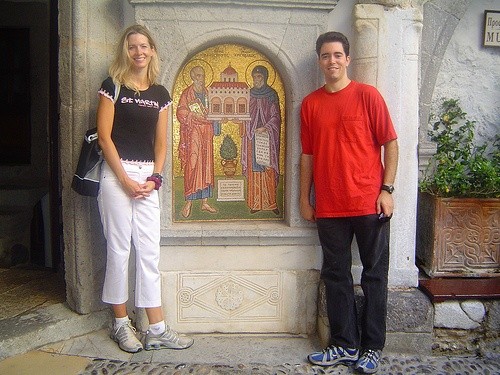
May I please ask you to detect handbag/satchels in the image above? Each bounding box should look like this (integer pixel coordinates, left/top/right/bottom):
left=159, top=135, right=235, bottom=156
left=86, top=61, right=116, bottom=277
left=71, top=127, right=103, bottom=196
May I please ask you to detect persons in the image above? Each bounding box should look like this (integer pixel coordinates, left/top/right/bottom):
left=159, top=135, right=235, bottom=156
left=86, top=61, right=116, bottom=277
left=96, top=24, right=193, bottom=354
left=299, top=31, right=399, bottom=374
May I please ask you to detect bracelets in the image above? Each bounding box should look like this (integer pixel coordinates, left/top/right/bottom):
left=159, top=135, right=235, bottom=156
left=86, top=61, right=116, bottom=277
left=147, top=173, right=163, bottom=191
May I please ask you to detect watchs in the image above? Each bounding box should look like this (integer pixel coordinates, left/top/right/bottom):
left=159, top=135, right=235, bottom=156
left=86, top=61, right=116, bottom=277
left=380, top=184, right=395, bottom=194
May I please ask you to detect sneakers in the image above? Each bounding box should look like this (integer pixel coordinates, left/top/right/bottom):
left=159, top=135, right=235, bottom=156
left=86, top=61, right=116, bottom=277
left=354, top=347, right=381, bottom=374
left=145, top=325, right=195, bottom=351
left=307, top=342, right=360, bottom=366
left=110, top=319, right=144, bottom=353
